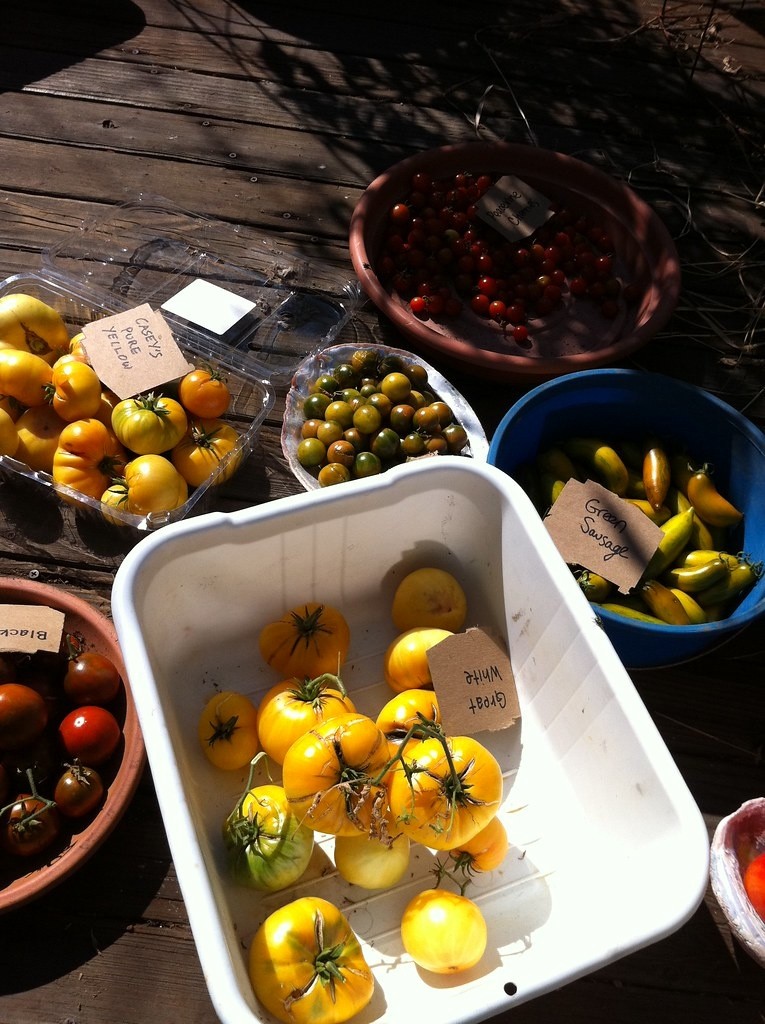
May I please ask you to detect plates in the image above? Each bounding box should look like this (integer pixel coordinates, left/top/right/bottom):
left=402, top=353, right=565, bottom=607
left=346, top=139, right=682, bottom=377
left=280, top=340, right=488, bottom=492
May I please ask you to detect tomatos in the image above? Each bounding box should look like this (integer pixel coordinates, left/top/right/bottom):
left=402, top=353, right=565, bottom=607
left=379, top=173, right=635, bottom=341
left=198, top=569, right=510, bottom=1022
left=1, top=291, right=240, bottom=521
left=1, top=632, right=119, bottom=851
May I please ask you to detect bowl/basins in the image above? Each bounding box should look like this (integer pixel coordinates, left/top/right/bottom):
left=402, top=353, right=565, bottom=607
left=482, top=367, right=764, bottom=663
left=0, top=572, right=140, bottom=914
left=713, top=794, right=765, bottom=971
left=110, top=451, right=713, bottom=1024
left=0, top=192, right=364, bottom=531
